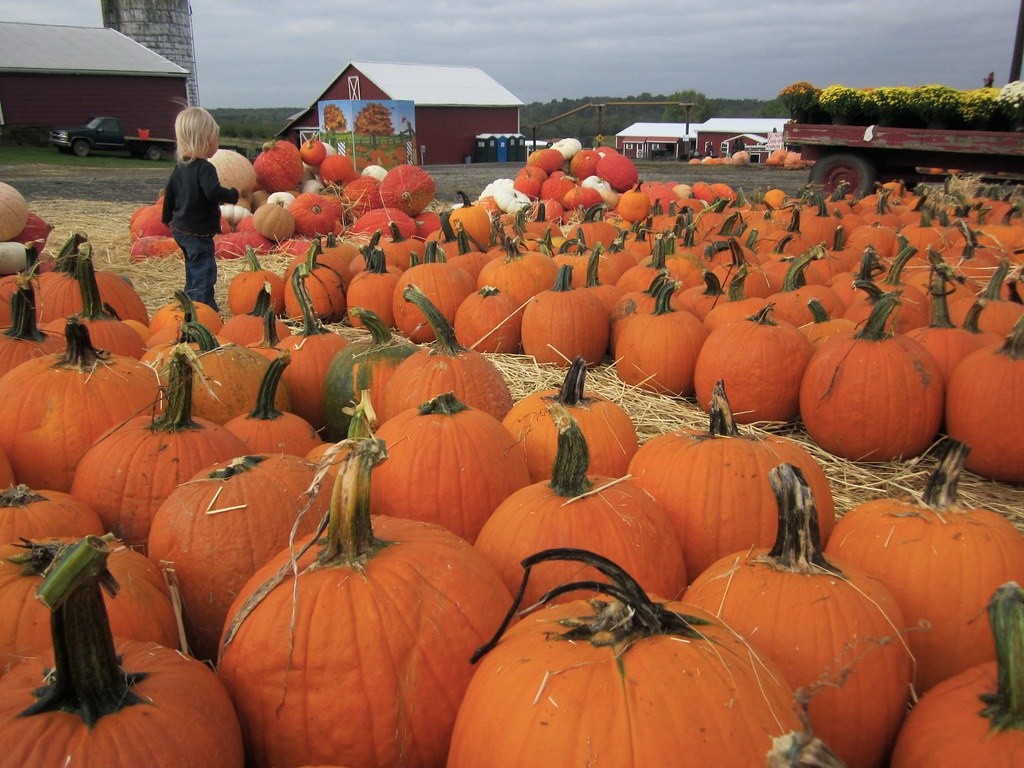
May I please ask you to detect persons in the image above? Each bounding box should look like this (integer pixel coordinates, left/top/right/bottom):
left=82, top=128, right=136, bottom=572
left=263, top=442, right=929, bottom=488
left=705, top=142, right=713, bottom=158
left=160, top=106, right=241, bottom=311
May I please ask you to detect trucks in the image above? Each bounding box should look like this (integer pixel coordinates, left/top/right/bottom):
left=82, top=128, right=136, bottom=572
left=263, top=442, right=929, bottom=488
left=777, top=122, right=1024, bottom=200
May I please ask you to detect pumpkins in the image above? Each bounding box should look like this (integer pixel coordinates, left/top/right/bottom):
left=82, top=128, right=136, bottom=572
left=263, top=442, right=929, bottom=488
left=128, top=138, right=1024, bottom=486
left=0, top=182, right=1024, bottom=768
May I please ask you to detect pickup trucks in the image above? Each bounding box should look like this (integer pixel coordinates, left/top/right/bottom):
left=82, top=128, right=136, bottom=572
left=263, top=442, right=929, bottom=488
left=47, top=114, right=177, bottom=161
